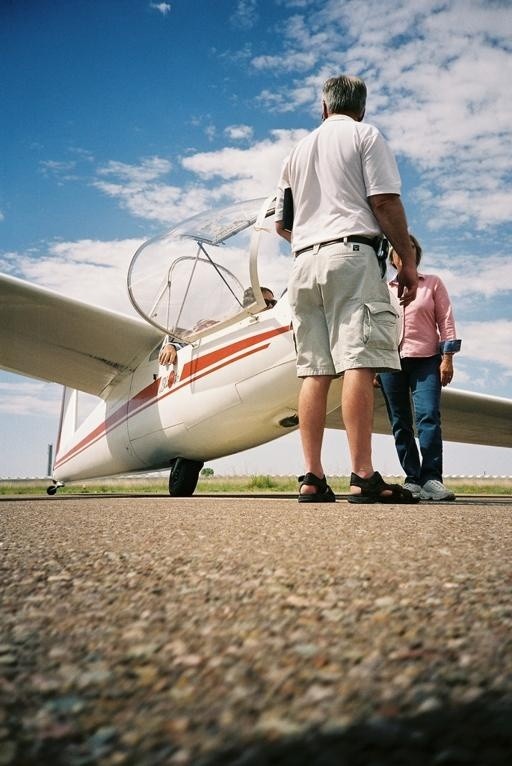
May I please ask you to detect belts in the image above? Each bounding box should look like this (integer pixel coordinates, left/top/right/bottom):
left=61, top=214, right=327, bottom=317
left=295, top=234, right=376, bottom=258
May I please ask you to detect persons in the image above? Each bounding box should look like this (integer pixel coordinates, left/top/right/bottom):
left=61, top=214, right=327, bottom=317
left=276, top=75, right=425, bottom=502
left=159, top=287, right=275, bottom=366
left=372, top=232, right=461, bottom=502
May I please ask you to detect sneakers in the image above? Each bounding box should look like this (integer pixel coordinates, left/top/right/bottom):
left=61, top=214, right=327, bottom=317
left=421, top=479, right=456, bottom=501
left=401, top=481, right=421, bottom=498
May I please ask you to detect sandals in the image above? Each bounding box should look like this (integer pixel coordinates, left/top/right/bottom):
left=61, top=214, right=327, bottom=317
left=298, top=473, right=336, bottom=502
left=346, top=471, right=420, bottom=505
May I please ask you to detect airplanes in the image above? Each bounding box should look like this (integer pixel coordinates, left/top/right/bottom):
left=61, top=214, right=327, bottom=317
left=0, top=193, right=512, bottom=499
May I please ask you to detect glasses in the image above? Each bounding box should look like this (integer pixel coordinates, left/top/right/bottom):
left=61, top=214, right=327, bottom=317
left=264, top=299, right=277, bottom=307
